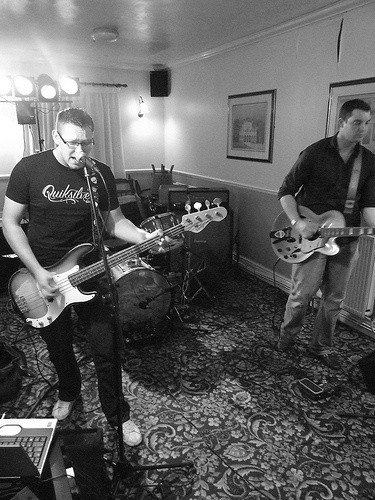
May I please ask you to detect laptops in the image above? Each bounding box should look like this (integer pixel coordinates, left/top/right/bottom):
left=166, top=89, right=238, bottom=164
left=0, top=418, right=57, bottom=481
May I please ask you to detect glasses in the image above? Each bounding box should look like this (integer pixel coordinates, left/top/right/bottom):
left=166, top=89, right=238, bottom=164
left=57, top=131, right=95, bottom=148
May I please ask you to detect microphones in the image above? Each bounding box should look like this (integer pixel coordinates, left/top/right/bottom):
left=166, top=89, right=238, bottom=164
left=76, top=152, right=100, bottom=173
left=139, top=301, right=151, bottom=309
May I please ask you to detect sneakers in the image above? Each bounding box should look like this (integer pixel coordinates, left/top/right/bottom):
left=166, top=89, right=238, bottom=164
left=52, top=391, right=81, bottom=420
left=115, top=419, right=142, bottom=446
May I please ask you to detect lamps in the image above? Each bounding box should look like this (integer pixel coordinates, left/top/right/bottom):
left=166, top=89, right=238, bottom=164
left=37, top=74, right=59, bottom=101
left=138, top=96, right=150, bottom=118
left=60, top=77, right=80, bottom=96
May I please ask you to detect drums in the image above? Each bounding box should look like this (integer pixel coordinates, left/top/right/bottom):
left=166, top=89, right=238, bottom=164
left=109, top=257, right=173, bottom=323
left=139, top=212, right=185, bottom=254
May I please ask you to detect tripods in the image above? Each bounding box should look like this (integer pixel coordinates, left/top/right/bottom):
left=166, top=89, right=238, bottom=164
left=84, top=164, right=193, bottom=500
left=178, top=234, right=215, bottom=313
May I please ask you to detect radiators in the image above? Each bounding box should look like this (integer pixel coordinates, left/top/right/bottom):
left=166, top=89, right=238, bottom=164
left=316, top=234, right=375, bottom=319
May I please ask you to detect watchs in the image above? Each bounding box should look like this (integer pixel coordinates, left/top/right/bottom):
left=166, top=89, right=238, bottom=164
left=291, top=219, right=297, bottom=226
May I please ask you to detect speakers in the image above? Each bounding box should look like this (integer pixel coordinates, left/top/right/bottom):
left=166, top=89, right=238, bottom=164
left=150, top=71, right=169, bottom=97
left=167, top=209, right=234, bottom=273
left=15, top=102, right=36, bottom=124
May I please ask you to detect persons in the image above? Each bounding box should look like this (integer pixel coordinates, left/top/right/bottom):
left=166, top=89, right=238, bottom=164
left=275, top=99, right=375, bottom=369
left=1, top=108, right=174, bottom=446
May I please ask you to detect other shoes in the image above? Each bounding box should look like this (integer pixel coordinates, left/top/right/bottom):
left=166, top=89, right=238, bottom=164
left=313, top=352, right=341, bottom=371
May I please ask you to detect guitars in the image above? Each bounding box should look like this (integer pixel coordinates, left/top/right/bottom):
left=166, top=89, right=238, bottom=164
left=7, top=197, right=227, bottom=329
left=268, top=205, right=374, bottom=264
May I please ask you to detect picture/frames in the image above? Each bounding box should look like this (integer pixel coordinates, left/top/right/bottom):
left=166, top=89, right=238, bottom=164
left=226, top=88, right=277, bottom=163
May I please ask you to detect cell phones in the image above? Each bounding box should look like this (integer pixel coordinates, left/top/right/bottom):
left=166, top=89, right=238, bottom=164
left=299, top=378, right=324, bottom=394
